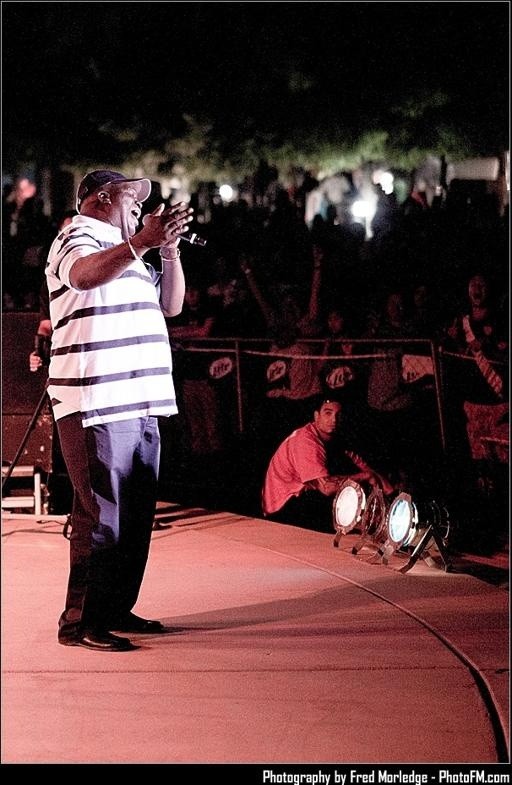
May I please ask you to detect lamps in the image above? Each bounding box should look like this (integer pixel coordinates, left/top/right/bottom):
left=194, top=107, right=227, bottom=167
left=330, top=472, right=455, bottom=577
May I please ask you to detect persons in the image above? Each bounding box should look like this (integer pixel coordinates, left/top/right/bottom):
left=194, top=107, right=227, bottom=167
left=2, top=167, right=508, bottom=651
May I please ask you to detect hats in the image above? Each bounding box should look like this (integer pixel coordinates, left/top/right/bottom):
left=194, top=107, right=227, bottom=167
left=76, top=169, right=152, bottom=215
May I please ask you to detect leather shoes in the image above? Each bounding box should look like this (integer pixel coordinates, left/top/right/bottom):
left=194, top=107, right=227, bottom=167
left=109, top=611, right=161, bottom=633
left=74, top=628, right=130, bottom=651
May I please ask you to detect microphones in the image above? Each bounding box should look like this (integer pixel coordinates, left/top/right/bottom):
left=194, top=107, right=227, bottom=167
left=142, top=214, right=207, bottom=247
